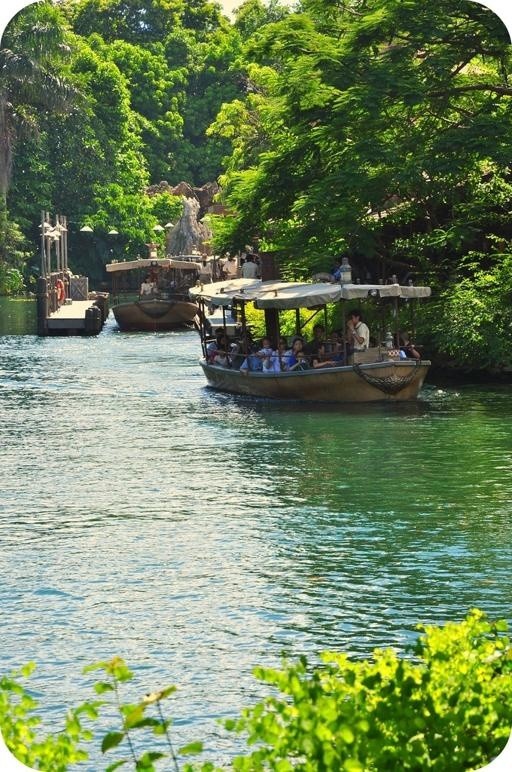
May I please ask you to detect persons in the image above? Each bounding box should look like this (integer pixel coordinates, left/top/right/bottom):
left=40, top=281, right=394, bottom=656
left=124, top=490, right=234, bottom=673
left=240, top=256, right=259, bottom=278
left=139, top=275, right=157, bottom=295
left=206, top=311, right=376, bottom=375
left=223, top=259, right=236, bottom=279
left=198, top=253, right=213, bottom=285
left=399, top=331, right=421, bottom=358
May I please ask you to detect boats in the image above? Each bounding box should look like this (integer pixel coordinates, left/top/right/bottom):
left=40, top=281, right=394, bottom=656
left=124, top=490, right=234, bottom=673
left=186, top=275, right=432, bottom=404
left=105, top=257, right=203, bottom=332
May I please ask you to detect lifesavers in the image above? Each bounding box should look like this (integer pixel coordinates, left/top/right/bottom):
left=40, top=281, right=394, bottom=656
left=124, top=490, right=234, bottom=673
left=57, top=280, right=65, bottom=301
